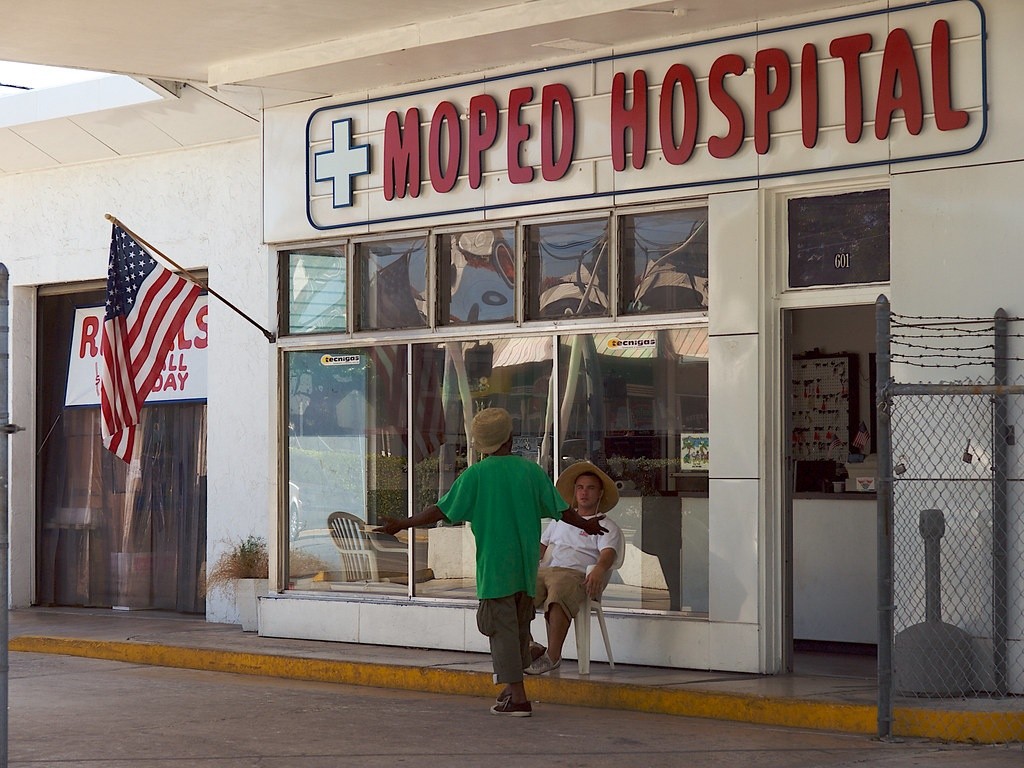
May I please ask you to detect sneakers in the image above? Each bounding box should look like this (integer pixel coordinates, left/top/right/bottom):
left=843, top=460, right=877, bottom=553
left=490, top=695, right=532, bottom=716
left=496, top=688, right=512, bottom=704
left=523, top=642, right=547, bottom=669
left=523, top=651, right=562, bottom=675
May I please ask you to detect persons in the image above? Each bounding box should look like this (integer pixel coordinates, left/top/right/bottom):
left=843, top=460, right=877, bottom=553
left=523, top=461, right=620, bottom=675
left=370, top=407, right=609, bottom=717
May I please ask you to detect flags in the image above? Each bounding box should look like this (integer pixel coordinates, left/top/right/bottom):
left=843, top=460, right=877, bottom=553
left=852, top=422, right=870, bottom=451
left=359, top=255, right=447, bottom=464
left=100, top=224, right=202, bottom=466
left=830, top=434, right=842, bottom=452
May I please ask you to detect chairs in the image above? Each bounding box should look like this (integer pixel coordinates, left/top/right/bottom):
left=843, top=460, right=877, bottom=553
left=574, top=528, right=626, bottom=674
left=327, top=511, right=408, bottom=583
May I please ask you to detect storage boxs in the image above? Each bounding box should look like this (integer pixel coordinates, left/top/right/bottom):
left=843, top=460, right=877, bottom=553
left=846, top=477, right=878, bottom=491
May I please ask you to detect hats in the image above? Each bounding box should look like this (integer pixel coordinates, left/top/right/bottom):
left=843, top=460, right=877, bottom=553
left=471, top=408, right=512, bottom=453
left=556, top=462, right=619, bottom=513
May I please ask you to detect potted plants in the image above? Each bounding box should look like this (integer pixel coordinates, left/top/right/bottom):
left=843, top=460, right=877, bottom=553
left=198, top=530, right=268, bottom=632
